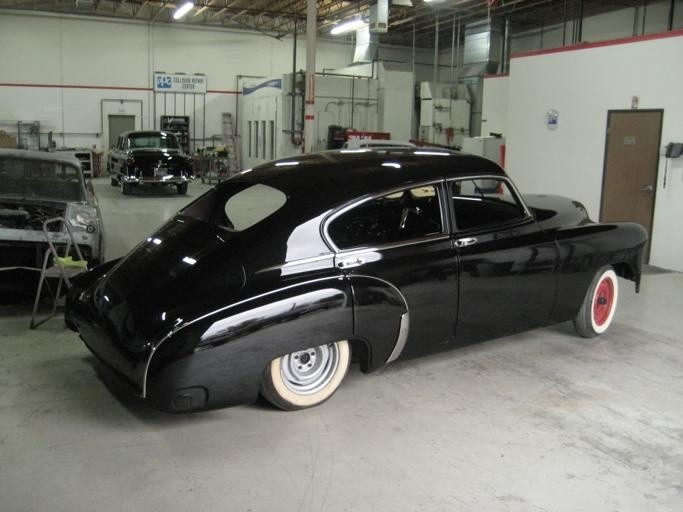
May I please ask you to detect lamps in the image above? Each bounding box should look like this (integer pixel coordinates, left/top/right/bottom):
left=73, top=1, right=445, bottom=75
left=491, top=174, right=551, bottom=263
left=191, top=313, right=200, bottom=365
left=330, top=16, right=370, bottom=36
left=171, top=1, right=194, bottom=22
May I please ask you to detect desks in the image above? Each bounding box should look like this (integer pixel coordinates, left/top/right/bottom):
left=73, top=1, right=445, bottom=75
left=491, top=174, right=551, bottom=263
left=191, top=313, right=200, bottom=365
left=200, top=158, right=229, bottom=185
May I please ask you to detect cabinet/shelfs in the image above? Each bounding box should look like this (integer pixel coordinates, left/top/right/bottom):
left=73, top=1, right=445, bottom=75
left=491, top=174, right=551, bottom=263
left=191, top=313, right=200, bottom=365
left=74, top=152, right=93, bottom=179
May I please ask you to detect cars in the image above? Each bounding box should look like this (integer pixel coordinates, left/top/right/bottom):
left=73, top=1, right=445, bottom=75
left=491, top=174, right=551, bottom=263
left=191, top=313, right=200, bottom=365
left=108, top=130, right=194, bottom=199
left=63, top=143, right=650, bottom=416
left=0, top=145, right=107, bottom=311
